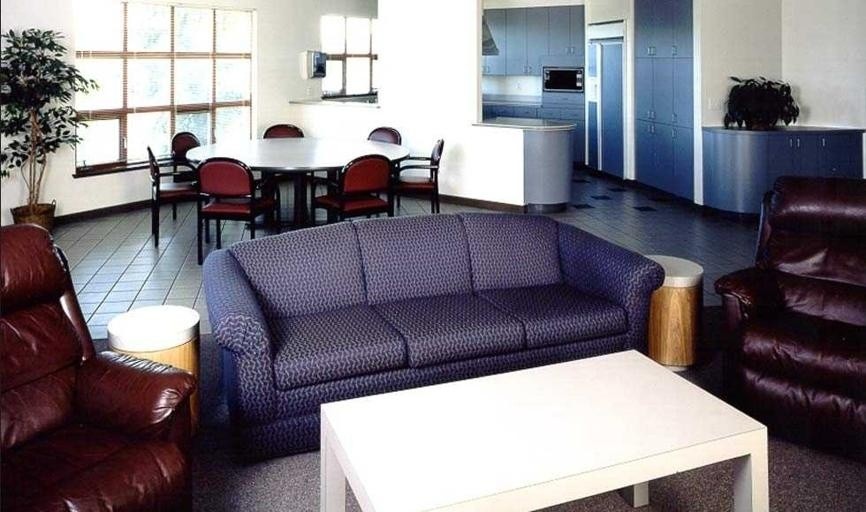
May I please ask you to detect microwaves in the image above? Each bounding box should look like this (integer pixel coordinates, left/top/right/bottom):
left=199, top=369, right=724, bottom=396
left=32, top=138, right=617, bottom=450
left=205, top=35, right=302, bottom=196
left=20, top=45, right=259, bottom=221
left=543, top=68, right=584, bottom=92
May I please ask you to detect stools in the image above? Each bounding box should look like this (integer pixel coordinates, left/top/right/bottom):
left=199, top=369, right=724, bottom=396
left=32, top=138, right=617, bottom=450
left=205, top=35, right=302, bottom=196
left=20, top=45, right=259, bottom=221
left=105, top=304, right=202, bottom=432
left=641, top=254, right=705, bottom=372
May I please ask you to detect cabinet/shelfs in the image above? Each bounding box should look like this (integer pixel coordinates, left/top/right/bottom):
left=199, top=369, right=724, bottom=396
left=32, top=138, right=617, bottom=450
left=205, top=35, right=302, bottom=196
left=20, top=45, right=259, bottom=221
left=549, top=7, right=584, bottom=56
left=484, top=105, right=537, bottom=120
left=635, top=119, right=673, bottom=196
left=672, top=60, right=694, bottom=127
left=702, top=128, right=864, bottom=215
left=635, top=58, right=671, bottom=125
left=506, top=8, right=548, bottom=75
left=538, top=109, right=584, bottom=164
left=481, top=10, right=505, bottom=75
left=671, top=1, right=692, bottom=59
left=635, top=1, right=672, bottom=57
left=673, top=127, right=695, bottom=200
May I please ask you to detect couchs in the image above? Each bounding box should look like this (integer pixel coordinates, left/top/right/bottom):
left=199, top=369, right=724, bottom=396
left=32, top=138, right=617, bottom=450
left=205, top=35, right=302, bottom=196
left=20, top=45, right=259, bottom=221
left=200, top=213, right=666, bottom=464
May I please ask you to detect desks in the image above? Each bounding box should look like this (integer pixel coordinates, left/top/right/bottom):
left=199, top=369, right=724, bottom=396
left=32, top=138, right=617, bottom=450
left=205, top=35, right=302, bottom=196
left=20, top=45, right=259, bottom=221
left=185, top=137, right=410, bottom=232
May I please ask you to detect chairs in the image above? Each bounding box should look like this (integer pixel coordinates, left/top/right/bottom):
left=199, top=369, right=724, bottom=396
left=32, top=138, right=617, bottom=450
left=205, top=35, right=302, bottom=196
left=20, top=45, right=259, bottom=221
left=1, top=220, right=197, bottom=512
left=712, top=177, right=865, bottom=455
left=308, top=154, right=396, bottom=226
left=171, top=131, right=201, bottom=221
left=395, top=139, right=445, bottom=214
left=260, top=124, right=307, bottom=215
left=194, top=157, right=279, bottom=267
left=144, top=146, right=210, bottom=248
left=368, top=127, right=403, bottom=218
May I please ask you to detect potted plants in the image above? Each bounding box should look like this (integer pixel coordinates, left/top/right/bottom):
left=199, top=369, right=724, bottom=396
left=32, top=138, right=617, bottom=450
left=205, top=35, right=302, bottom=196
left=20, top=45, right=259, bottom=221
left=723, top=76, right=799, bottom=130
left=0, top=27, right=101, bottom=234
